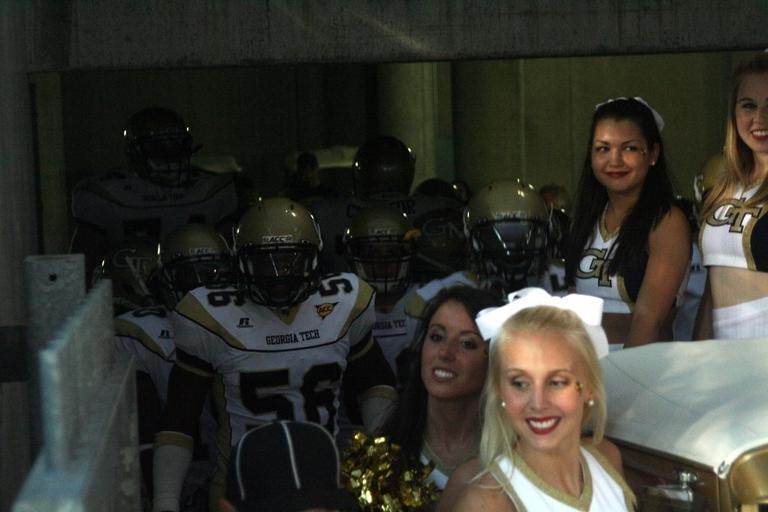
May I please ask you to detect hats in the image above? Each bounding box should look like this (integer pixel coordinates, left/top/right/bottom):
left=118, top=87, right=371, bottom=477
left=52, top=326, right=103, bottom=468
left=223, top=419, right=353, bottom=512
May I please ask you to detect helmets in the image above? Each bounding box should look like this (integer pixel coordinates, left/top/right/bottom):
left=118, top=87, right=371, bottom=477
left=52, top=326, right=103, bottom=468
left=406, top=179, right=466, bottom=265
left=462, top=181, right=551, bottom=285
left=231, top=197, right=323, bottom=310
left=90, top=240, right=177, bottom=313
left=351, top=135, right=415, bottom=201
left=341, top=192, right=414, bottom=306
left=121, top=108, right=194, bottom=187
left=142, top=225, right=233, bottom=312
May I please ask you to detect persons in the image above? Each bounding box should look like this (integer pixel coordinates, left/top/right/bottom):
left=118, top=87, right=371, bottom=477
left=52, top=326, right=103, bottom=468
left=568, top=97, right=690, bottom=354
left=400, top=283, right=639, bottom=511
left=71, top=107, right=575, bottom=512
left=692, top=54, right=766, bottom=342
